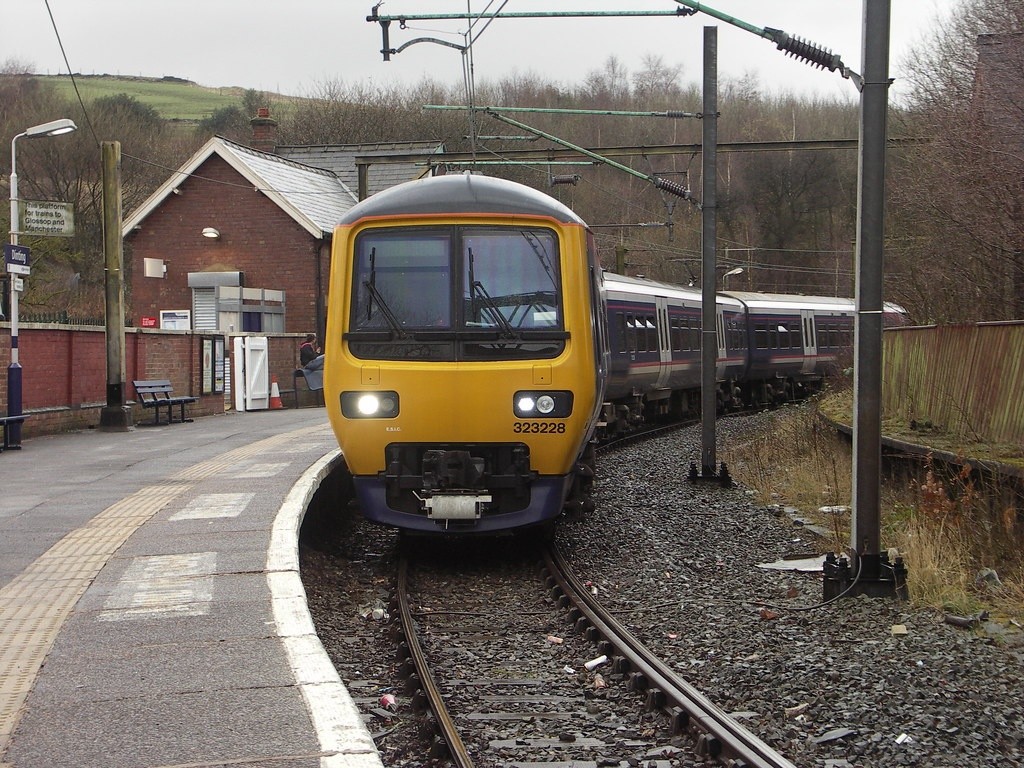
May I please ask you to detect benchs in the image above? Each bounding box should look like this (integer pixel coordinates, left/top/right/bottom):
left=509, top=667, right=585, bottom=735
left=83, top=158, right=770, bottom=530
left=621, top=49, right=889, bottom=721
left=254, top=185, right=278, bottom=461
left=133, top=379, right=196, bottom=426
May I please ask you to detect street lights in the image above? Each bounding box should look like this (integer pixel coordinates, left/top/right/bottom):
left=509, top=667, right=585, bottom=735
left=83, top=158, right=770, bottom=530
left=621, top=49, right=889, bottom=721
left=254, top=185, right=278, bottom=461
left=2, top=118, right=76, bottom=456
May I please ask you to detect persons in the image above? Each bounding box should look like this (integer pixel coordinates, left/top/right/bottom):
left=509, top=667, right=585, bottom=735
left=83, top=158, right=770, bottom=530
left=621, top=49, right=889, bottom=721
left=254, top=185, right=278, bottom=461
left=300, top=334, right=321, bottom=367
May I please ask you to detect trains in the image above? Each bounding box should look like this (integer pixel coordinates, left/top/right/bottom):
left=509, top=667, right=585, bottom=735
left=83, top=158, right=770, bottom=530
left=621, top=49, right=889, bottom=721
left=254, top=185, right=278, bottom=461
left=325, top=170, right=926, bottom=560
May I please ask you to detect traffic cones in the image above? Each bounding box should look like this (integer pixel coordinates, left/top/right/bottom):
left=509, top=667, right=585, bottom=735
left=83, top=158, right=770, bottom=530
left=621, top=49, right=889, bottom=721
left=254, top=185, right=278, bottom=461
left=265, top=375, right=287, bottom=410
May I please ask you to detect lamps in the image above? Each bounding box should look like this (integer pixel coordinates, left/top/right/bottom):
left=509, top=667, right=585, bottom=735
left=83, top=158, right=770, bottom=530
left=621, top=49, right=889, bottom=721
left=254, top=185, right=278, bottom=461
left=202, top=227, right=220, bottom=238
